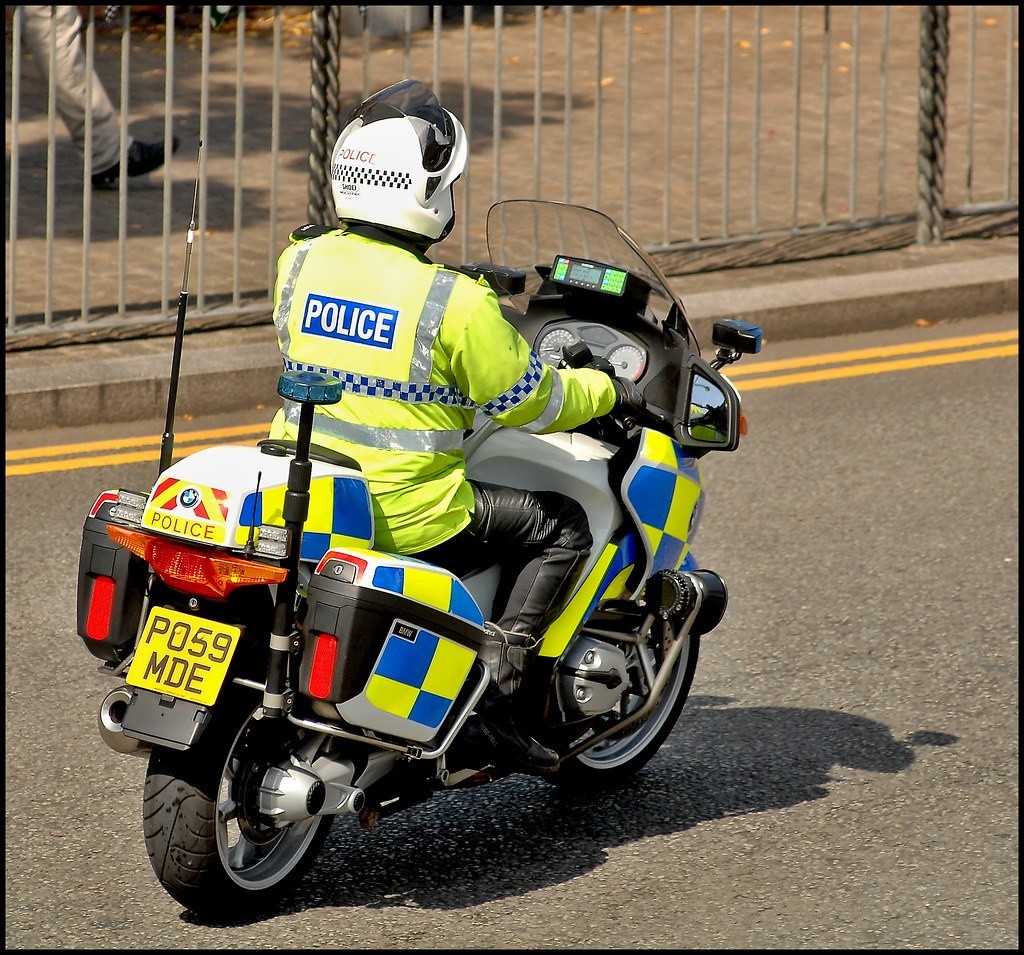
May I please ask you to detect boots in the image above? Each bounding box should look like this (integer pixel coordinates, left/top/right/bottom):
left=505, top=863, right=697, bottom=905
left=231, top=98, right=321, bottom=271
left=463, top=619, right=560, bottom=775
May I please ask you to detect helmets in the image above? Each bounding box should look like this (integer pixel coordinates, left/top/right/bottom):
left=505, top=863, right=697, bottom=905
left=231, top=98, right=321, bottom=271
left=330, top=104, right=469, bottom=246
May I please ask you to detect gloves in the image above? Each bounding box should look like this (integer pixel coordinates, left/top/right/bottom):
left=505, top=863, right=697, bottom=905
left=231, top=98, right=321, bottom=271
left=610, top=376, right=646, bottom=414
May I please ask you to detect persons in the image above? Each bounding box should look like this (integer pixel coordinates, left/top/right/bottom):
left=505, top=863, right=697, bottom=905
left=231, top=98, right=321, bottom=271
left=268, top=79, right=643, bottom=772
left=11, top=6, right=180, bottom=192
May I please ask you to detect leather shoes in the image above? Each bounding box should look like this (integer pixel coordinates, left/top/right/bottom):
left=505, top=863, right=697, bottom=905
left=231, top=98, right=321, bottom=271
left=91, top=137, right=178, bottom=188
